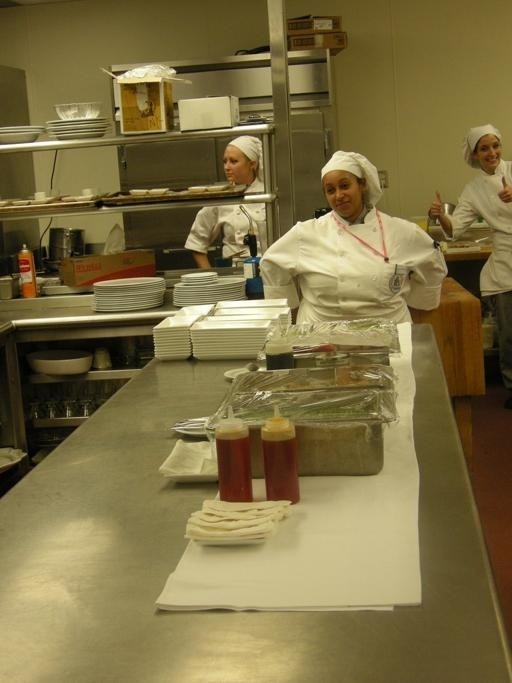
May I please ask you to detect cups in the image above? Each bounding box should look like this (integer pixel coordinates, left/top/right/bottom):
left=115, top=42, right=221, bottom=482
left=92, top=346, right=113, bottom=369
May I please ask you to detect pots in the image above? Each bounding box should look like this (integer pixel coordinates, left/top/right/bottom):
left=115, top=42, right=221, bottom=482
left=47, top=227, right=85, bottom=261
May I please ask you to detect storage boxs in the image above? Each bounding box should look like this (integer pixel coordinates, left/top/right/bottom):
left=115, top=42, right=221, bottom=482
left=178, top=95, right=239, bottom=132
left=99, top=64, right=192, bottom=135
left=59, top=247, right=156, bottom=289
left=287, top=13, right=348, bottom=53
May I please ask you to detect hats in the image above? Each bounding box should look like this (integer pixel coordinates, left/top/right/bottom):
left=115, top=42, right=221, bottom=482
left=229, top=135, right=264, bottom=183
left=462, top=123, right=503, bottom=169
left=320, top=150, right=383, bottom=210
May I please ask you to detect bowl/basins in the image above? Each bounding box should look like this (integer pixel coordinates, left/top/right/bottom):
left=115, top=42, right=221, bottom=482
left=54, top=101, right=102, bottom=120
left=25, top=350, right=93, bottom=375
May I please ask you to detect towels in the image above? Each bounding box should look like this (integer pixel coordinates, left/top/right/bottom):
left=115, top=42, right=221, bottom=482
left=152, top=317, right=424, bottom=612
left=158, top=440, right=218, bottom=479
left=185, top=498, right=294, bottom=543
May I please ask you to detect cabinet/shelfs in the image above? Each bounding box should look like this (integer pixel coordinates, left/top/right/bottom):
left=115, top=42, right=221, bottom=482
left=0, top=122, right=277, bottom=247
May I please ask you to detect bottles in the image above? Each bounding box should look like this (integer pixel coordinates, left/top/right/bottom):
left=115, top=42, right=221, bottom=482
left=481, top=312, right=497, bottom=349
left=261, top=404, right=301, bottom=506
left=265, top=329, right=295, bottom=369
left=17, top=244, right=38, bottom=298
left=214, top=405, right=253, bottom=503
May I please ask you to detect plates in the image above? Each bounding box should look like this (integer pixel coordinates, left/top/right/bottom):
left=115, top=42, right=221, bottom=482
left=0, top=195, right=56, bottom=206
left=174, top=417, right=208, bottom=438
left=0, top=126, right=46, bottom=144
left=152, top=298, right=293, bottom=363
left=224, top=366, right=266, bottom=379
left=172, top=271, right=248, bottom=307
left=46, top=117, right=112, bottom=140
left=187, top=183, right=231, bottom=192
left=160, top=438, right=218, bottom=484
left=129, top=187, right=169, bottom=196
left=0, top=447, right=28, bottom=474
left=42, top=285, right=91, bottom=295
left=92, top=276, right=166, bottom=312
left=61, top=194, right=102, bottom=201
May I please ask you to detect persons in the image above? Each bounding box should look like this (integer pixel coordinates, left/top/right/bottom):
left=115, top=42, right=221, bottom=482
left=184, top=132, right=268, bottom=269
left=259, top=149, right=447, bottom=328
left=429, top=122, right=512, bottom=412
